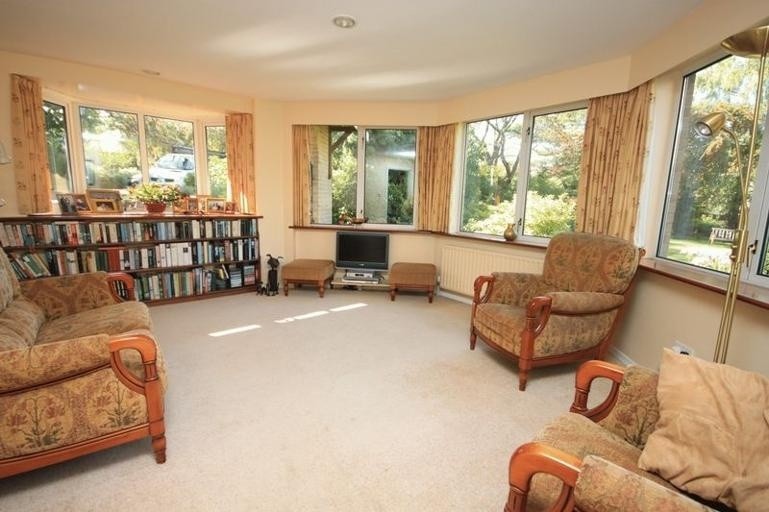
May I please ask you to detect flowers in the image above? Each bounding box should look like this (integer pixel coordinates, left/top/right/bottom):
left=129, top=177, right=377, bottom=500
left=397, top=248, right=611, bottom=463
left=127, top=182, right=183, bottom=203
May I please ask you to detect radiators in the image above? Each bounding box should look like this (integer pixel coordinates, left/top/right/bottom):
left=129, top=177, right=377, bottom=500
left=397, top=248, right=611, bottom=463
left=439, top=244, right=545, bottom=300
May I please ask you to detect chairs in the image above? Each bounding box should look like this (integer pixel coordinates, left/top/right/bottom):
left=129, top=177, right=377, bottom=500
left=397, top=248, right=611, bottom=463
left=503, top=361, right=768, bottom=512
left=470, top=231, right=647, bottom=391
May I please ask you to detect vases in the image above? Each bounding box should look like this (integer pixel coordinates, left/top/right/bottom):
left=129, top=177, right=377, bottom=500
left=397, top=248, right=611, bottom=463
left=144, top=200, right=166, bottom=213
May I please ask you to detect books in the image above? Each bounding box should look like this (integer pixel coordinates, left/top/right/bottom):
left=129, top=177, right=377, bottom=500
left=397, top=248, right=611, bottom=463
left=1, top=217, right=259, bottom=303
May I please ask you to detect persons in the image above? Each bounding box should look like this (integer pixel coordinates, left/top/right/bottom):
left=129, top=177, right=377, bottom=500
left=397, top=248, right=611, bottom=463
left=215, top=203, right=221, bottom=210
left=96, top=202, right=107, bottom=212
left=212, top=204, right=218, bottom=210
left=60, top=197, right=76, bottom=214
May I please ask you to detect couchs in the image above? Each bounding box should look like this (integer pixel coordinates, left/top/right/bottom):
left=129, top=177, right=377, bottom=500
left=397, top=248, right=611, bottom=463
left=0, top=247, right=170, bottom=479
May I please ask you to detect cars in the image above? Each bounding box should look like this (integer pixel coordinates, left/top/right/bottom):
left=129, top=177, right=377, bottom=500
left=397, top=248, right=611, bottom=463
left=128, top=173, right=143, bottom=185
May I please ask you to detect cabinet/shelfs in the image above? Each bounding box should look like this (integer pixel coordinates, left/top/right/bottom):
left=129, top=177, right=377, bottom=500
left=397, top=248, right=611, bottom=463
left=1, top=213, right=268, bottom=309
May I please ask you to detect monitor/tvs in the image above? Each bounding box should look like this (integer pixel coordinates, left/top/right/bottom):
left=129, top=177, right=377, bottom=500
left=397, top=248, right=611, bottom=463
left=336, top=232, right=390, bottom=272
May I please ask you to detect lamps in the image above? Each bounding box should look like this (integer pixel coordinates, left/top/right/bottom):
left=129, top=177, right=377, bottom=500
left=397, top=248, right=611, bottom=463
left=695, top=22, right=768, bottom=362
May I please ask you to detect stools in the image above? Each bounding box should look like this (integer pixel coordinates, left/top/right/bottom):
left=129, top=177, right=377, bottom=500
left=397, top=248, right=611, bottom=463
left=280, top=257, right=335, bottom=297
left=391, top=263, right=436, bottom=302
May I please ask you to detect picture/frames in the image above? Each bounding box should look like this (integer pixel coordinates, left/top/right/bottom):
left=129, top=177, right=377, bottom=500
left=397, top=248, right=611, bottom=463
left=56, top=188, right=123, bottom=215
left=172, top=195, right=235, bottom=214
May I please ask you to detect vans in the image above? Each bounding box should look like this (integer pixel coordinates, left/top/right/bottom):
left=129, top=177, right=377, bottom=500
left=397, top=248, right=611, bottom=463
left=148, top=154, right=195, bottom=188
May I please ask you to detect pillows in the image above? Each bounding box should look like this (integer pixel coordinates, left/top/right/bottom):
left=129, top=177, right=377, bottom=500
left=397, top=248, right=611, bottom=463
left=637, top=346, right=768, bottom=512
